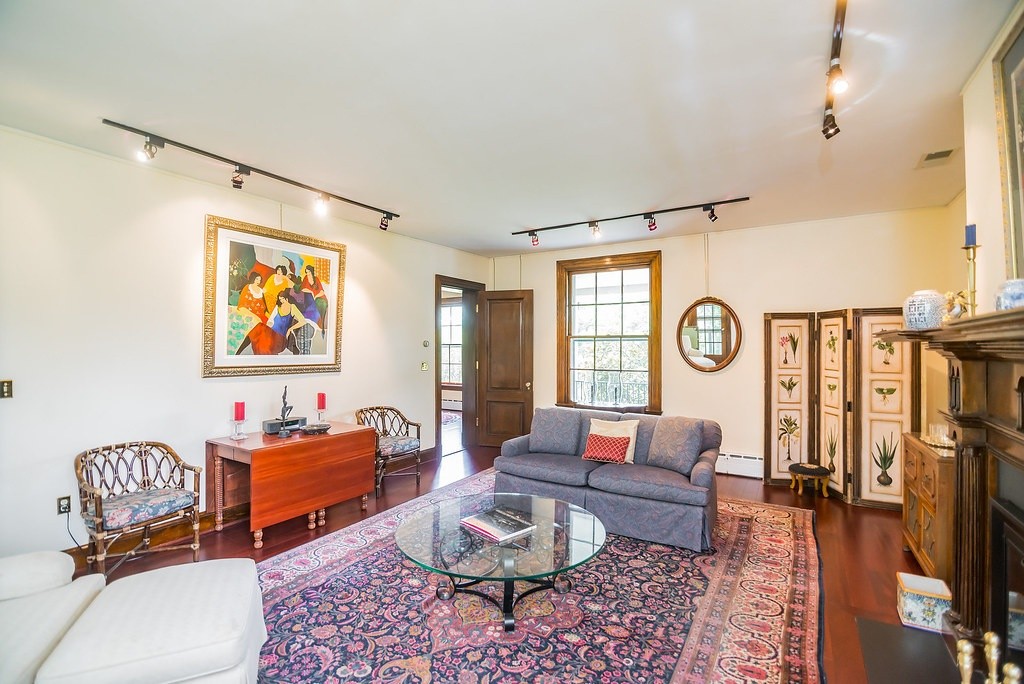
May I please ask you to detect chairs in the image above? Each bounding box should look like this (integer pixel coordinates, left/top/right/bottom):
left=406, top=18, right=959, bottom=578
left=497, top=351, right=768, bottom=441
left=0, top=548, right=270, bottom=684
left=73, top=441, right=202, bottom=579
left=355, top=406, right=423, bottom=499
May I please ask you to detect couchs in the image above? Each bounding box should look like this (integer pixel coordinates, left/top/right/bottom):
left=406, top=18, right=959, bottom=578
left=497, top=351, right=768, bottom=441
left=493, top=409, right=724, bottom=553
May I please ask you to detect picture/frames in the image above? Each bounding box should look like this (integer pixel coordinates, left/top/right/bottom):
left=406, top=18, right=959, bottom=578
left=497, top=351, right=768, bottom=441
left=200, top=215, right=348, bottom=378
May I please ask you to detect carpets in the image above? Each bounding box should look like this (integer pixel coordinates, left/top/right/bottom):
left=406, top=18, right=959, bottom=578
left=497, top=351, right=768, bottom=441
left=855, top=616, right=961, bottom=684
left=256, top=465, right=828, bottom=684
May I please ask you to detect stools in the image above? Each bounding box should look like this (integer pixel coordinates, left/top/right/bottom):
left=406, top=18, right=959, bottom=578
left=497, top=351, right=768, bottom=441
left=788, top=462, right=832, bottom=500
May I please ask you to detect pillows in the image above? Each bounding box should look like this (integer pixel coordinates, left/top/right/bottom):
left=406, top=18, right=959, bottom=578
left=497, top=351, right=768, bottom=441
left=582, top=434, right=631, bottom=466
left=646, top=416, right=705, bottom=478
left=527, top=406, right=582, bottom=456
left=582, top=417, right=640, bottom=465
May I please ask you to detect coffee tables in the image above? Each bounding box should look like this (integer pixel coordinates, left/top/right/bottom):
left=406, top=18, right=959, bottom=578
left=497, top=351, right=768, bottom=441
left=393, top=492, right=607, bottom=633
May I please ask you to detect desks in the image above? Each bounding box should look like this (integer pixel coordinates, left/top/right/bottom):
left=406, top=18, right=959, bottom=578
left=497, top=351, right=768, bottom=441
left=204, top=420, right=376, bottom=550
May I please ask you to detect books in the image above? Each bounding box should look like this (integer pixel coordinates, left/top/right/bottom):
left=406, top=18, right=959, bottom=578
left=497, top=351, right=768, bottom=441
left=460, top=509, right=537, bottom=542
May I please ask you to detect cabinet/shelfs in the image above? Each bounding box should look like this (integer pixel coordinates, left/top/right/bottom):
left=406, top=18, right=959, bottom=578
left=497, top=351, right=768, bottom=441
left=901, top=431, right=956, bottom=580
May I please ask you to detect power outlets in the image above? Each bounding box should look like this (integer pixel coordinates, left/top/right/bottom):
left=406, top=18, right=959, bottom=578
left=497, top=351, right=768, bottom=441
left=57, top=496, right=70, bottom=515
left=0, top=380, right=12, bottom=398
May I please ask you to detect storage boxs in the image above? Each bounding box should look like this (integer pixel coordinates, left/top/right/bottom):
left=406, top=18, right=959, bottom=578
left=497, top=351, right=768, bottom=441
left=1008, top=591, right=1024, bottom=652
left=894, top=571, right=956, bottom=636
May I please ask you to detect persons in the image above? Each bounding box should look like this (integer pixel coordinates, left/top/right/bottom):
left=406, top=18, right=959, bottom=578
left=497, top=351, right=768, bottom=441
left=276, top=386, right=292, bottom=420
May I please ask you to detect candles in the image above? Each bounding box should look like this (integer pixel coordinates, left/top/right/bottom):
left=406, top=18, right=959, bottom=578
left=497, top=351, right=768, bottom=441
left=964, top=224, right=977, bottom=246
left=317, top=393, right=326, bottom=409
left=234, top=402, right=246, bottom=421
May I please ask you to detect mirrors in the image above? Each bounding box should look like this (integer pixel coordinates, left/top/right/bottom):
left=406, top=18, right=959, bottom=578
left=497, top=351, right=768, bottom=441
left=676, top=296, right=742, bottom=374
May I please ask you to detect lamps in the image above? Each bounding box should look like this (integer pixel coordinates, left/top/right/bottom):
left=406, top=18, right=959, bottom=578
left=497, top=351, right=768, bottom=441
left=828, top=67, right=848, bottom=94
left=703, top=205, right=718, bottom=223
left=135, top=137, right=165, bottom=162
left=643, top=213, right=657, bottom=232
left=589, top=222, right=601, bottom=238
left=230, top=166, right=251, bottom=189
left=528, top=231, right=539, bottom=246
left=379, top=213, right=393, bottom=231
left=313, top=194, right=330, bottom=218
left=822, top=114, right=841, bottom=140
